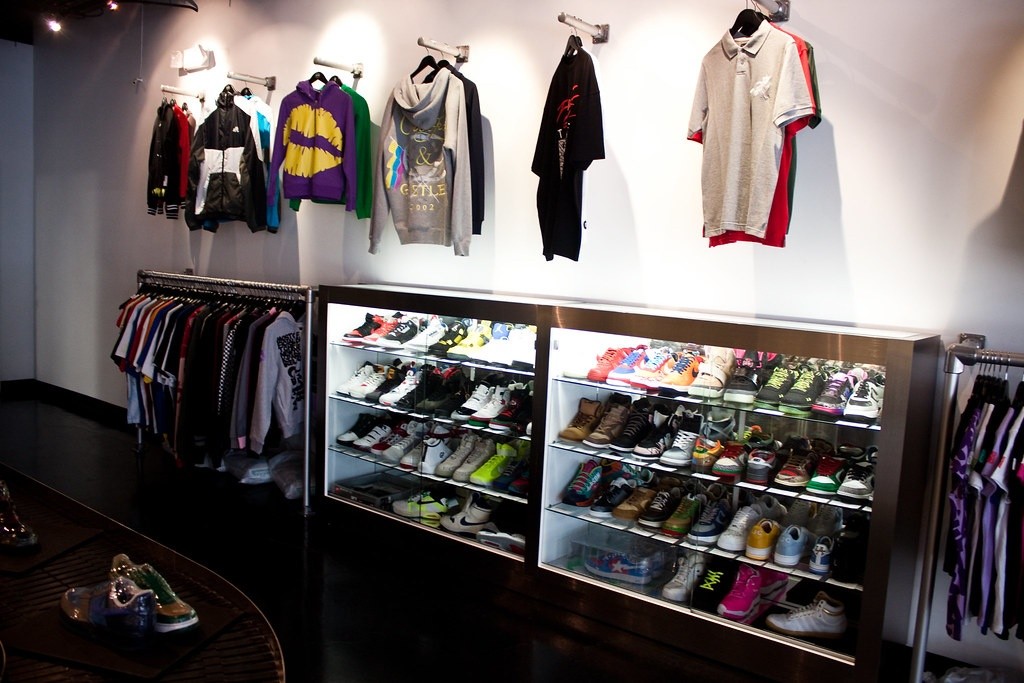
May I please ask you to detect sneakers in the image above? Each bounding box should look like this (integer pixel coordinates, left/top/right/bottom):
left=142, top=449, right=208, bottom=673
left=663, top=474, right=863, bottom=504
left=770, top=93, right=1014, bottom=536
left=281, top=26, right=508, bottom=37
left=746, top=516, right=786, bottom=561
left=339, top=360, right=533, bottom=552
left=719, top=563, right=789, bottom=619
left=561, top=455, right=678, bottom=522
left=341, top=305, right=536, bottom=370
left=810, top=533, right=837, bottom=575
left=809, top=503, right=843, bottom=534
left=109, top=554, right=198, bottom=632
left=686, top=496, right=734, bottom=546
left=686, top=555, right=742, bottom=610
left=782, top=499, right=819, bottom=527
left=716, top=501, right=764, bottom=552
left=657, top=493, right=704, bottom=538
left=753, top=493, right=786, bottom=521
left=584, top=543, right=671, bottom=584
left=638, top=490, right=680, bottom=527
left=774, top=524, right=815, bottom=566
left=62, top=578, right=156, bottom=631
left=556, top=344, right=886, bottom=500
left=660, top=552, right=707, bottom=601
left=766, top=591, right=847, bottom=635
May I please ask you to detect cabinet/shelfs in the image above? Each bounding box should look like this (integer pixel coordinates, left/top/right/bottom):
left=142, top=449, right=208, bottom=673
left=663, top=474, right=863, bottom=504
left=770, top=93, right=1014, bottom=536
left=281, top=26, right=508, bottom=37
left=311, top=284, right=939, bottom=683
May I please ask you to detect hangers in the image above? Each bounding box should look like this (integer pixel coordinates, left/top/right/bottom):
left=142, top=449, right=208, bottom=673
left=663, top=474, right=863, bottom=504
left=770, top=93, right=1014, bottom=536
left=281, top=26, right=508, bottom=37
left=308, top=57, right=343, bottom=88
left=224, top=73, right=254, bottom=98
left=730, top=0, right=769, bottom=36
left=160, top=86, right=190, bottom=113
left=970, top=351, right=1024, bottom=409
left=119, top=275, right=306, bottom=322
left=410, top=39, right=451, bottom=83
left=562, top=14, right=584, bottom=57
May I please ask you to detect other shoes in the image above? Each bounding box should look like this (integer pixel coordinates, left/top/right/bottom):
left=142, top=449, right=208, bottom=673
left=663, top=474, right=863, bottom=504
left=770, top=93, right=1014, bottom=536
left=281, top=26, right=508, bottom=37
left=0, top=480, right=38, bottom=545
left=705, top=483, right=732, bottom=502
left=827, top=511, right=871, bottom=581
left=679, top=477, right=705, bottom=493
